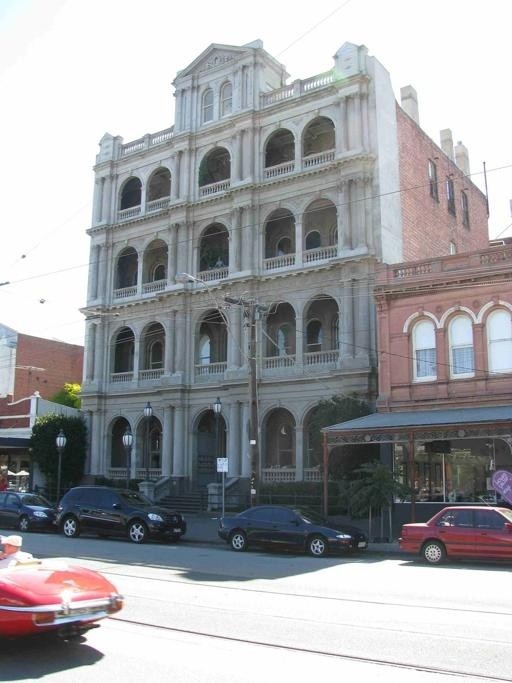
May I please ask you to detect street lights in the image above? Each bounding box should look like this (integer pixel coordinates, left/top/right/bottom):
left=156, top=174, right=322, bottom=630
left=143, top=401, right=152, bottom=481
left=122, top=425, right=133, bottom=488
left=174, top=271, right=262, bottom=507
left=213, top=397, right=221, bottom=483
left=55, top=428, right=67, bottom=507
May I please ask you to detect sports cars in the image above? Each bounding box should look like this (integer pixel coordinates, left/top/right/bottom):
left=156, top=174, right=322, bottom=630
left=1, top=536, right=123, bottom=641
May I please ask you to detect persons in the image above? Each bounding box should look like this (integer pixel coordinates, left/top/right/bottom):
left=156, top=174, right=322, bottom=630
left=214, top=255, right=226, bottom=269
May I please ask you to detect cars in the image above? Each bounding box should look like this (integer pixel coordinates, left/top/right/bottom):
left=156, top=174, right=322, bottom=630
left=399, top=505, right=512, bottom=564
left=218, top=503, right=369, bottom=557
left=55, top=485, right=186, bottom=544
left=0, top=489, right=59, bottom=531
